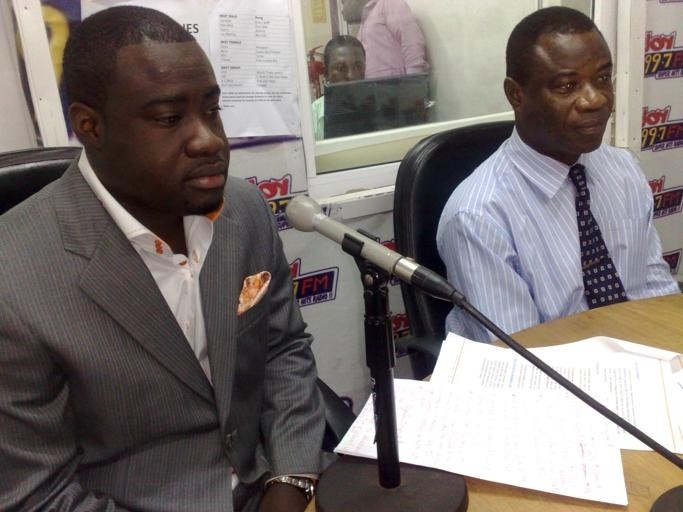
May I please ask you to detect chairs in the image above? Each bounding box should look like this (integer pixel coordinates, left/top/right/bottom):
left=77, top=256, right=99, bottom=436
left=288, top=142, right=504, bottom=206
left=0, top=145, right=358, bottom=466
left=392, top=120, right=519, bottom=380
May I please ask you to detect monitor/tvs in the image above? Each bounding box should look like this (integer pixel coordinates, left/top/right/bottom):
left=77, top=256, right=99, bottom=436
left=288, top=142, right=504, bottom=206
left=323, top=72, right=431, bottom=140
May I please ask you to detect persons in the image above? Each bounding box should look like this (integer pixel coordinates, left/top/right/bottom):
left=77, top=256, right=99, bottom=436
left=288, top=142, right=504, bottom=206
left=0, top=7, right=329, bottom=512
left=437, top=5, right=682, bottom=342
left=341, top=0, right=430, bottom=80
left=311, top=35, right=366, bottom=141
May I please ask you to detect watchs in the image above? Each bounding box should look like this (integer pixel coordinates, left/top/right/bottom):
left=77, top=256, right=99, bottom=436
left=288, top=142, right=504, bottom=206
left=267, top=475, right=314, bottom=501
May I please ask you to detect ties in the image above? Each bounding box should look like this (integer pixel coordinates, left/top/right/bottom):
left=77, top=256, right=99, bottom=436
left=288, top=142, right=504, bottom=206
left=568, top=164, right=627, bottom=310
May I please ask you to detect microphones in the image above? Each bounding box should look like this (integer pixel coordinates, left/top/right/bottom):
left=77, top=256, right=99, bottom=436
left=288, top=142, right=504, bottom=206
left=285, top=194, right=466, bottom=312
